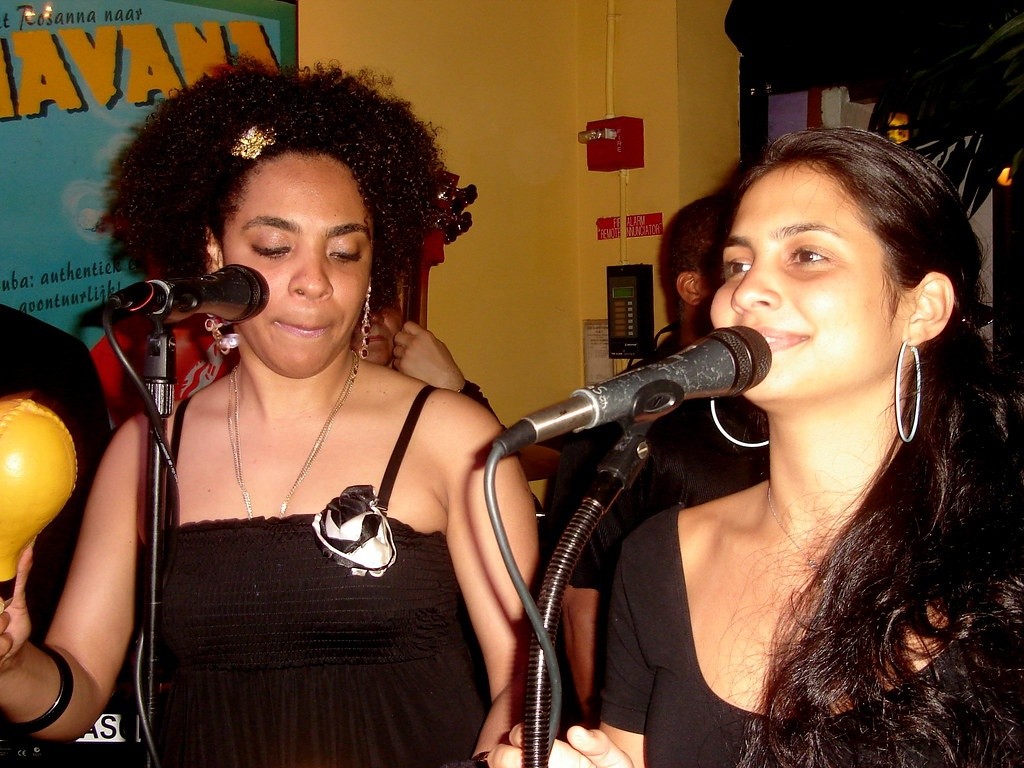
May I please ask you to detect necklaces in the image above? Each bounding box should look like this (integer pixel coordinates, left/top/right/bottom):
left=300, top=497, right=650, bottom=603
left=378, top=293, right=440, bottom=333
left=216, top=346, right=362, bottom=519
left=766, top=486, right=825, bottom=571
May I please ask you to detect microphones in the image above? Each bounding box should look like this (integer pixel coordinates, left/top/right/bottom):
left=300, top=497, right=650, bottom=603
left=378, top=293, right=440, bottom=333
left=491, top=326, right=771, bottom=460
left=110, top=263, right=271, bottom=324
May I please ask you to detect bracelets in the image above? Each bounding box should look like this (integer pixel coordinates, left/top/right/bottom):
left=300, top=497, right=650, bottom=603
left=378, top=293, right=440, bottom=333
left=12, top=634, right=75, bottom=736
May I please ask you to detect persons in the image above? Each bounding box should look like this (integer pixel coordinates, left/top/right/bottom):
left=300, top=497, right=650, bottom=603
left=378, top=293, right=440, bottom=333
left=354, top=302, right=502, bottom=420
left=545, top=129, right=1023, bottom=767
left=1, top=52, right=542, bottom=767
